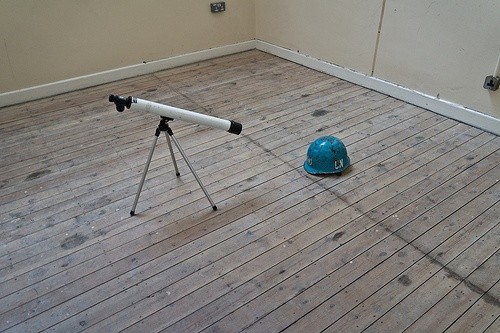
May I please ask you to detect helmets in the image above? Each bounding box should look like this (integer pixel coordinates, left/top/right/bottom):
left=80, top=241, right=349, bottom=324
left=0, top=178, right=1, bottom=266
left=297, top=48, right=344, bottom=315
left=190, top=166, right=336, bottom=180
left=303, top=135, right=350, bottom=174
